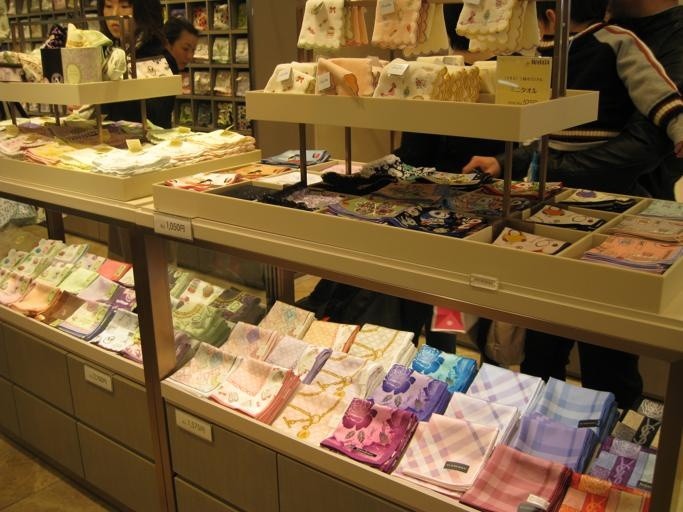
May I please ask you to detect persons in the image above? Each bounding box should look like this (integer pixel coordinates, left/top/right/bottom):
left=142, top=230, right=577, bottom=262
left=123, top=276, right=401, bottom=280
left=94, top=0, right=181, bottom=128
left=462, top=2, right=682, bottom=422
left=164, top=17, right=200, bottom=70
left=395, top=0, right=520, bottom=369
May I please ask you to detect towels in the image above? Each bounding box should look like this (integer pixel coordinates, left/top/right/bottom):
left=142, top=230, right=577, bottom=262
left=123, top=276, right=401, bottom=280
left=152, top=1, right=682, bottom=316
left=0, top=1, right=256, bottom=200
left=162, top=297, right=664, bottom=512
left=0, top=233, right=260, bottom=382
